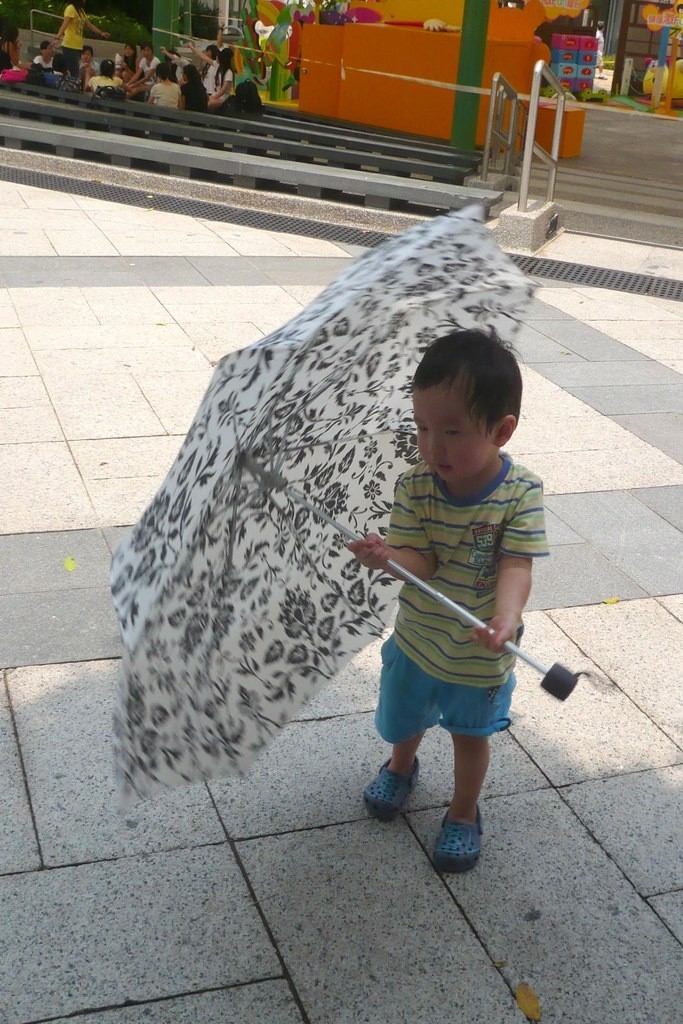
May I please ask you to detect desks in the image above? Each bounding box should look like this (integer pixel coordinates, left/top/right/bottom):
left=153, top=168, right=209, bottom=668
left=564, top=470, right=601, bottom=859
left=337, top=23, right=536, bottom=155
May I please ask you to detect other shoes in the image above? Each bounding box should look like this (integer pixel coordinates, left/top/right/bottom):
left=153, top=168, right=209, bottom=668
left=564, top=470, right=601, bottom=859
left=435, top=803, right=483, bottom=871
left=364, top=755, right=420, bottom=817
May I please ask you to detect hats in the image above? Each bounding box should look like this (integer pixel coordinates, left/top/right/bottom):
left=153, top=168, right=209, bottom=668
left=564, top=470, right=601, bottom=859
left=101, top=60, right=115, bottom=73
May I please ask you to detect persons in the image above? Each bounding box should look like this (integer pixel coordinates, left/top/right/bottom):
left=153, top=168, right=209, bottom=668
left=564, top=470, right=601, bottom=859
left=594, top=22, right=610, bottom=80
left=0, top=0, right=267, bottom=118
left=340, top=320, right=545, bottom=872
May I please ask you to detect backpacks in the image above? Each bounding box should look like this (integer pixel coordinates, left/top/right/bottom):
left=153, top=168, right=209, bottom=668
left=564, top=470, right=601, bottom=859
left=235, top=79, right=264, bottom=113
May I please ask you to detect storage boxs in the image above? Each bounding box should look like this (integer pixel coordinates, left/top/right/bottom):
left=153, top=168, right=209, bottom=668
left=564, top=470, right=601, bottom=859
left=551, top=33, right=598, bottom=95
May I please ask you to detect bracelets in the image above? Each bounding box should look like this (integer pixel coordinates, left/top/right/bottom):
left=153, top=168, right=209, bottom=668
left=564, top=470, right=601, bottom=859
left=100, top=31, right=102, bottom=35
left=55, top=38, right=60, bottom=40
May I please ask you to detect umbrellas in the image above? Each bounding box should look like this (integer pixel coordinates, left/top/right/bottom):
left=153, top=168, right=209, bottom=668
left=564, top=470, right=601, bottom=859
left=109, top=203, right=582, bottom=799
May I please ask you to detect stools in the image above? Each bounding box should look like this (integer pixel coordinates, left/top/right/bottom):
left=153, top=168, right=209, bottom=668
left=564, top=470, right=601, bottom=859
left=534, top=104, right=585, bottom=159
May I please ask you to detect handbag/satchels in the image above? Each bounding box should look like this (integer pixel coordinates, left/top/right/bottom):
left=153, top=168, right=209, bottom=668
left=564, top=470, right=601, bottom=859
left=92, top=85, right=127, bottom=101
